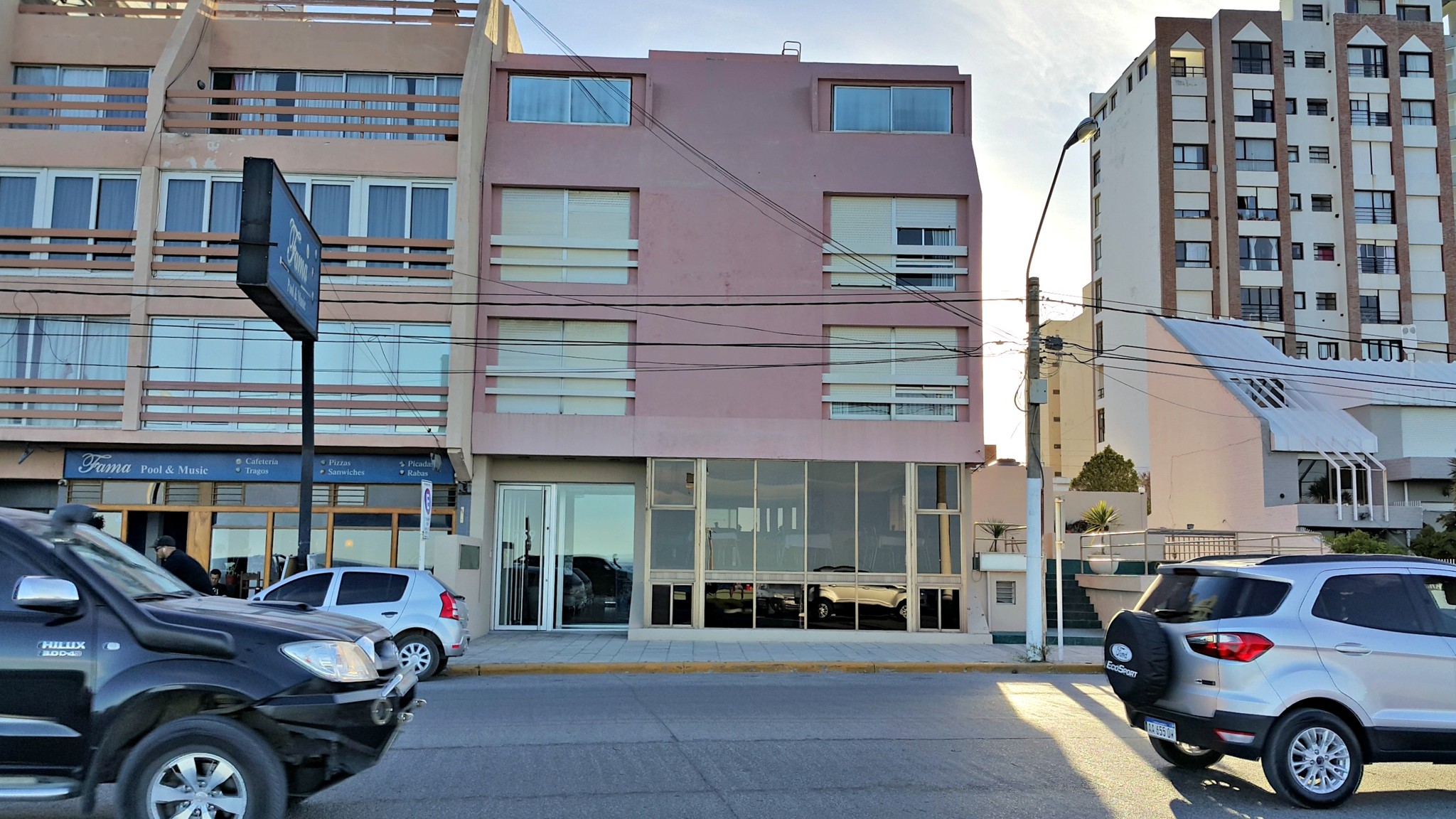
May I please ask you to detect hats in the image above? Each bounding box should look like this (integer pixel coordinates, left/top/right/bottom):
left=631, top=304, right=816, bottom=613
left=148, top=536, right=176, bottom=549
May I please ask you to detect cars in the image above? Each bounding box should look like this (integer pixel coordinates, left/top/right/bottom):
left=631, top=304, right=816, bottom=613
left=501, top=554, right=920, bottom=625
left=245, top=565, right=469, bottom=678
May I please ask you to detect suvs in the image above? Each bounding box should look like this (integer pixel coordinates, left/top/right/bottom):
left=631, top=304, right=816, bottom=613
left=1, top=505, right=416, bottom=818
left=1104, top=553, right=1454, bottom=808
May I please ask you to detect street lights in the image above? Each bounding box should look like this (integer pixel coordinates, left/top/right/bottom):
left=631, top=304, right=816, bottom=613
left=1025, top=117, right=1100, bottom=664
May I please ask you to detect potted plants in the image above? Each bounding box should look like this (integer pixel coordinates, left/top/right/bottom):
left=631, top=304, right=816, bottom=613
left=1078, top=498, right=1126, bottom=575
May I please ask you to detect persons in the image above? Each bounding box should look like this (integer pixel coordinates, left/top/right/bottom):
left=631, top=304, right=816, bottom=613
left=147, top=535, right=216, bottom=597
left=205, top=568, right=234, bottom=598
left=705, top=581, right=753, bottom=626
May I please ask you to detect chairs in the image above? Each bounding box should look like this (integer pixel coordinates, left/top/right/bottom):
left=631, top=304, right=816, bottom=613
left=1323, top=582, right=1446, bottom=637
left=236, top=569, right=262, bottom=601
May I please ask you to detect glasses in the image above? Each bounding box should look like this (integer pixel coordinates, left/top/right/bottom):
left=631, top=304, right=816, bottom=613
left=154, top=545, right=164, bottom=552
